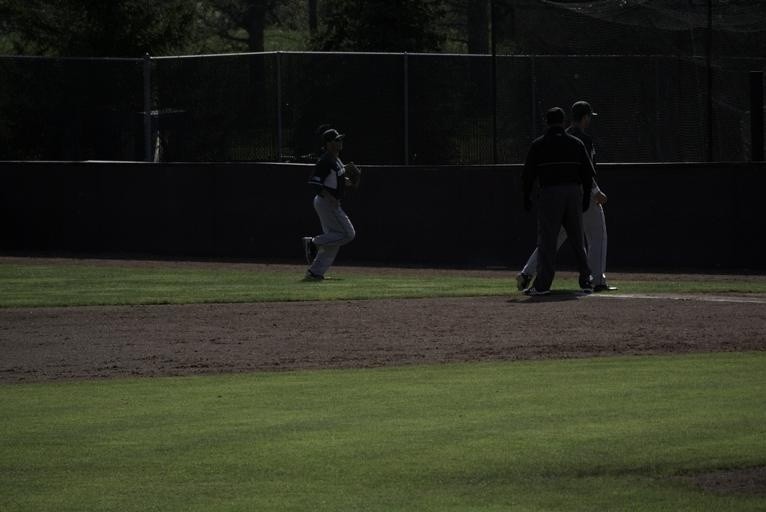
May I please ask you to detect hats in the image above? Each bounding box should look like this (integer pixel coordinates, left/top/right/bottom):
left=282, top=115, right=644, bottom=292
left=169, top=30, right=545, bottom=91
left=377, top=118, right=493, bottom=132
left=546, top=107, right=565, bottom=125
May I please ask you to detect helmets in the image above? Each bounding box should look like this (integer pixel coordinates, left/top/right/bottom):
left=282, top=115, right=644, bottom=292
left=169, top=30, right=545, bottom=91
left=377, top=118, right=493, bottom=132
left=571, top=101, right=599, bottom=118
left=322, top=129, right=346, bottom=144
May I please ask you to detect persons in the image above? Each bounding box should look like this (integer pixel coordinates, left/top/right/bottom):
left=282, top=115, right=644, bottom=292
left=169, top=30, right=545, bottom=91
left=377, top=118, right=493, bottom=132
left=304, top=128, right=359, bottom=281
left=521, top=107, right=596, bottom=297
left=515, top=100, right=619, bottom=291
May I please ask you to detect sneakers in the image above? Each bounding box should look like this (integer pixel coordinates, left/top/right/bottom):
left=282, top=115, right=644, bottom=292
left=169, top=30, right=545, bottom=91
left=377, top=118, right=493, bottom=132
left=514, top=274, right=619, bottom=297
left=302, top=235, right=325, bottom=281
